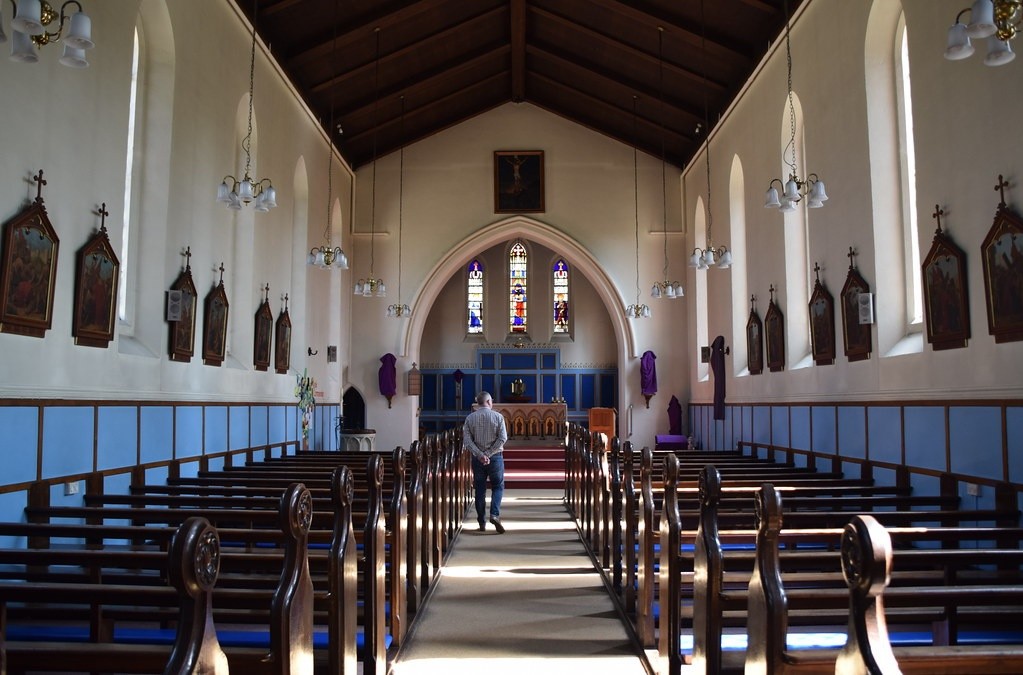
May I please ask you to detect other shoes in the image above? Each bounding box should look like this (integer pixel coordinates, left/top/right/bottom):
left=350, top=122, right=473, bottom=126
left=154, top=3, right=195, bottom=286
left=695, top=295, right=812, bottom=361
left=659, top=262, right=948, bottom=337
left=480, top=524, right=485, bottom=531
left=490, top=516, right=505, bottom=533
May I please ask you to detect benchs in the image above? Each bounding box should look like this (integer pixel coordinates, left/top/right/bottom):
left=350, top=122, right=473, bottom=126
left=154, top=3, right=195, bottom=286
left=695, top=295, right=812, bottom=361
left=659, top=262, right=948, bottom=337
left=562, top=420, right=1023, bottom=675
left=0, top=424, right=475, bottom=675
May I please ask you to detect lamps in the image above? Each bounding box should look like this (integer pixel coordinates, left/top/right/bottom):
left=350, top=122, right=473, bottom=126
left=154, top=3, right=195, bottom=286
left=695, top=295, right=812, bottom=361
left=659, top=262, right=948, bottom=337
left=384, top=95, right=412, bottom=319
left=0, top=0, right=97, bottom=69
left=689, top=0, right=733, bottom=270
left=763, top=0, right=828, bottom=213
left=353, top=27, right=387, bottom=298
left=944, top=0, right=1023, bottom=65
left=649, top=24, right=686, bottom=299
left=305, top=0, right=350, bottom=272
left=215, top=0, right=278, bottom=215
left=624, top=95, right=652, bottom=320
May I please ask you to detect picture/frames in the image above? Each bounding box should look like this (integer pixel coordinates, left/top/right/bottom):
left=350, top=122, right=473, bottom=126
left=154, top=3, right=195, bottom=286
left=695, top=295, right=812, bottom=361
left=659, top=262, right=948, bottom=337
left=494, top=150, right=546, bottom=214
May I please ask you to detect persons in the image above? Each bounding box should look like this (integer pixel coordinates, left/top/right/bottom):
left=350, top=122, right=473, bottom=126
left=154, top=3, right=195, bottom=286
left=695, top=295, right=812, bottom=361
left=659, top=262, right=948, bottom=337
left=461, top=390, right=507, bottom=534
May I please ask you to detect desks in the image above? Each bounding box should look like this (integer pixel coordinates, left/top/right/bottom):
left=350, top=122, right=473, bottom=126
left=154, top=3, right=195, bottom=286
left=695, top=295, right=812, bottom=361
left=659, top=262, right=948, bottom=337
left=471, top=403, right=568, bottom=440
